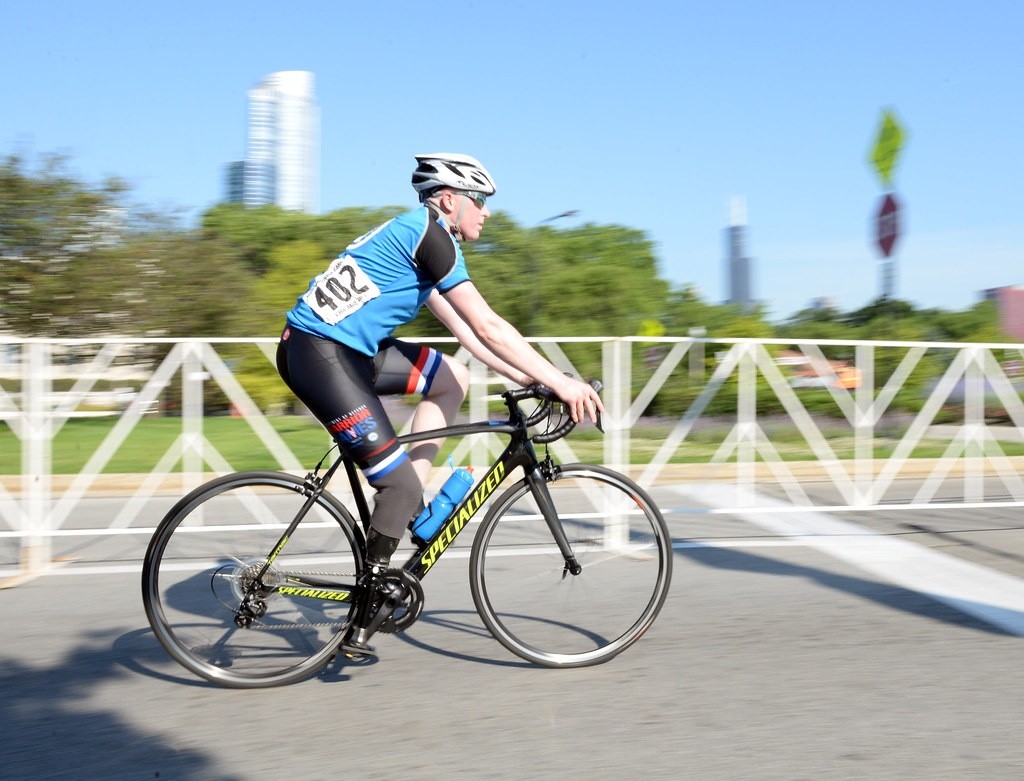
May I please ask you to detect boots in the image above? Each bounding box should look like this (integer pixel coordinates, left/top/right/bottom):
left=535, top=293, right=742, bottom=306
left=356, top=526, right=403, bottom=601
left=407, top=497, right=426, bottom=548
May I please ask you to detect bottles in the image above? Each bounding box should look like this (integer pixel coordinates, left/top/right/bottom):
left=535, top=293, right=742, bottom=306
left=412, top=464, right=474, bottom=543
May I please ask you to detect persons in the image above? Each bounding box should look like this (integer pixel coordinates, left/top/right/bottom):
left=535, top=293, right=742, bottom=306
left=275, top=154, right=606, bottom=653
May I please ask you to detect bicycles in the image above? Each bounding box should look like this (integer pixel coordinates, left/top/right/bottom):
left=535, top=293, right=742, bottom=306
left=142, top=373, right=674, bottom=690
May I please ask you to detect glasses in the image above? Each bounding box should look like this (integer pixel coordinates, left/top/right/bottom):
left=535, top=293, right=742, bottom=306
left=452, top=191, right=488, bottom=209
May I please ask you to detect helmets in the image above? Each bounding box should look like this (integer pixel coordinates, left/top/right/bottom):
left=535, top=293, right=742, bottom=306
left=411, top=152, right=497, bottom=201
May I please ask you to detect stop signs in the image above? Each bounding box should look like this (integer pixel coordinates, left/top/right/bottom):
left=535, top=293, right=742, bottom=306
left=878, top=196, right=896, bottom=255
